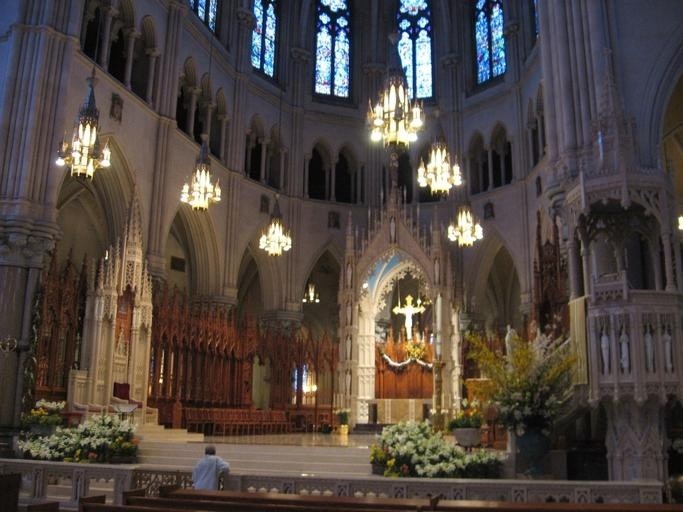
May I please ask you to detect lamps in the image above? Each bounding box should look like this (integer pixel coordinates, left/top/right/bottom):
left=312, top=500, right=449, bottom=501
left=55, top=0, right=113, bottom=180
left=179, top=2, right=293, bottom=259
left=364, top=0, right=484, bottom=247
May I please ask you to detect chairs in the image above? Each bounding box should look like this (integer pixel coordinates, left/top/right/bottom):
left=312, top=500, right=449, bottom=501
left=183, top=406, right=297, bottom=439
left=66, top=373, right=159, bottom=429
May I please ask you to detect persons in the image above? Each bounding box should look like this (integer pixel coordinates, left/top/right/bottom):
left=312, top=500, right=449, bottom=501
left=192, top=446, right=230, bottom=491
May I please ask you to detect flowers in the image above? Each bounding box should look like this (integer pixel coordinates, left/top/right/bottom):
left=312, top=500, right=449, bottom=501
left=332, top=406, right=351, bottom=424
left=368, top=323, right=587, bottom=478
left=15, top=397, right=142, bottom=465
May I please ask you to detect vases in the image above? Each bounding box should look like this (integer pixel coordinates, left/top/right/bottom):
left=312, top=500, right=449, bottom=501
left=339, top=424, right=349, bottom=436
